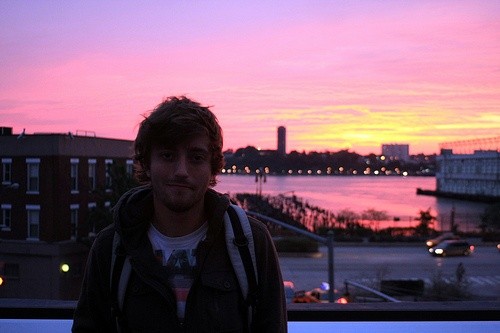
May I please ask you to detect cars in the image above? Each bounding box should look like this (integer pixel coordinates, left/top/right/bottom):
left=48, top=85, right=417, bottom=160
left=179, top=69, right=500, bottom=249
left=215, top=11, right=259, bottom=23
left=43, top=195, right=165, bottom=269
left=306, top=283, right=346, bottom=303
left=426, top=233, right=474, bottom=256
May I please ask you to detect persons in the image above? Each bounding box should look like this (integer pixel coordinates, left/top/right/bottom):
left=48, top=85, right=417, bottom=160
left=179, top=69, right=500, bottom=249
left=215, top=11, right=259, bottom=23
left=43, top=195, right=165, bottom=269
left=72, top=96, right=288, bottom=333
